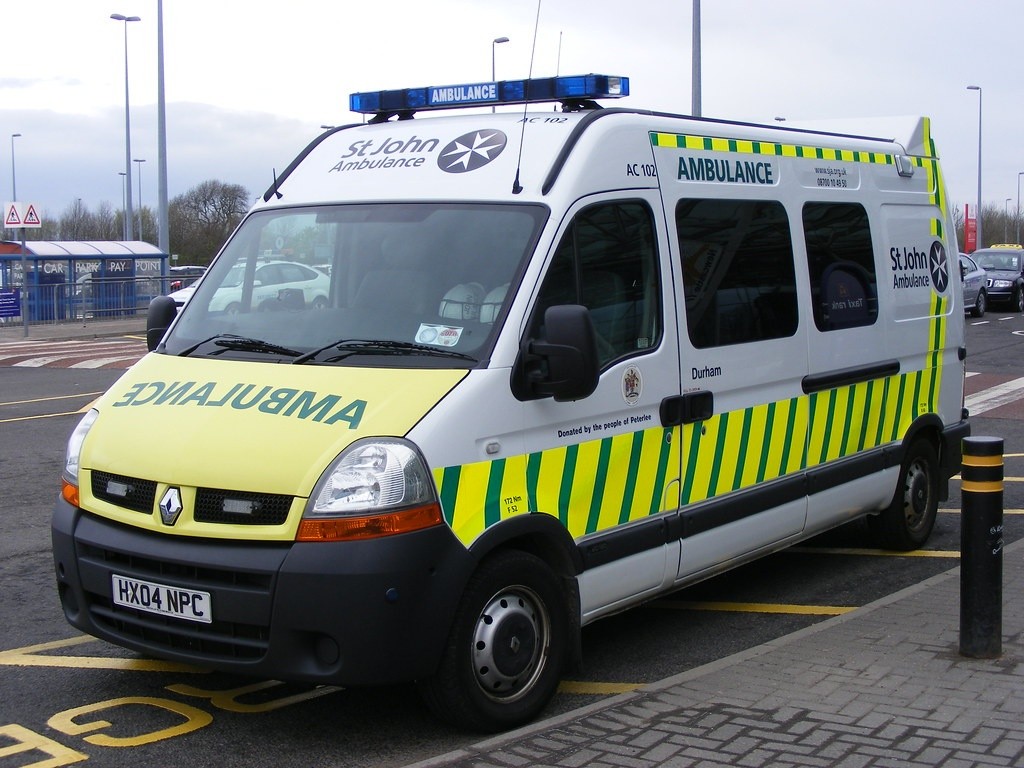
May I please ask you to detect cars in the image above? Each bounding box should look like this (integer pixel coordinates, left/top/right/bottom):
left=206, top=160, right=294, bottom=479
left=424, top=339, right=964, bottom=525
left=959, top=253, right=988, bottom=318
left=970, top=244, right=1024, bottom=313
left=166, top=256, right=332, bottom=317
left=152, top=266, right=207, bottom=294
left=136, top=275, right=159, bottom=295
left=64, top=273, right=93, bottom=299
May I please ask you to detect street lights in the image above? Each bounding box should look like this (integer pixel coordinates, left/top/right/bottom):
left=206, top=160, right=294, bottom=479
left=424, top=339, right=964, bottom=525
left=111, top=14, right=146, bottom=240
left=493, top=37, right=508, bottom=112
left=12, top=134, right=21, bottom=240
left=134, top=159, right=146, bottom=242
left=967, top=85, right=983, bottom=251
left=1017, top=172, right=1024, bottom=245
left=119, top=172, right=128, bottom=242
left=1005, top=198, right=1012, bottom=244
left=79, top=198, right=81, bottom=217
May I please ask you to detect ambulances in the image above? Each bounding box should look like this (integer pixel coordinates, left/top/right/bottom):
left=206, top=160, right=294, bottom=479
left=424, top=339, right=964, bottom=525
left=50, top=76, right=969, bottom=733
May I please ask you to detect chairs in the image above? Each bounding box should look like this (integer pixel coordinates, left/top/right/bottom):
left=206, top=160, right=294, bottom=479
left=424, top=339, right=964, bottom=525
left=568, top=269, right=628, bottom=357
left=352, top=235, right=430, bottom=314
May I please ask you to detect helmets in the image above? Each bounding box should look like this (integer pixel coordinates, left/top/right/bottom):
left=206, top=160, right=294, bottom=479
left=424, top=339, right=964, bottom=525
left=437, top=282, right=511, bottom=325
